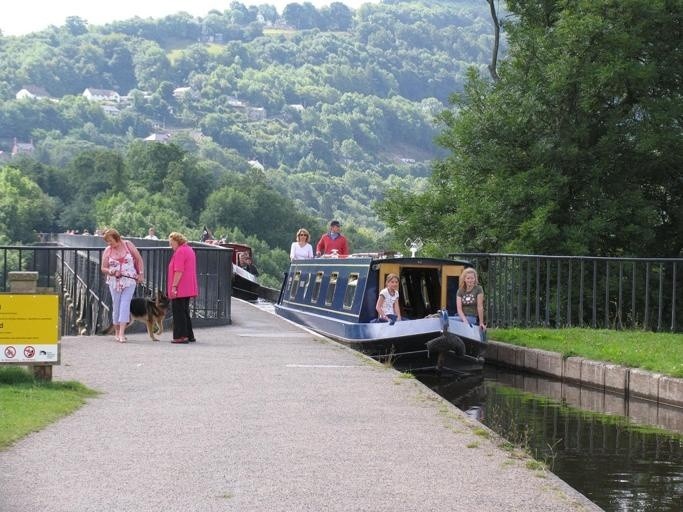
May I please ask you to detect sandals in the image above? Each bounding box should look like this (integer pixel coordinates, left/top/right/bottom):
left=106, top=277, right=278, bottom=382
left=171, top=336, right=196, bottom=343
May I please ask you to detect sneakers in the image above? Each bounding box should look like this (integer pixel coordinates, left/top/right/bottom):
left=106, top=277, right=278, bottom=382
left=116, top=336, right=127, bottom=343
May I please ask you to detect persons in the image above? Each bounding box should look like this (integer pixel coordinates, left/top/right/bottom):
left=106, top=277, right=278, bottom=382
left=68, top=230, right=74, bottom=234
left=373, top=273, right=410, bottom=323
left=143, top=227, right=159, bottom=239
left=315, top=221, right=349, bottom=256
left=243, top=258, right=259, bottom=277
left=74, top=230, right=79, bottom=234
left=81, top=229, right=89, bottom=235
left=100, top=228, right=144, bottom=343
left=165, top=231, right=199, bottom=344
left=447, top=267, right=486, bottom=332
left=289, top=228, right=313, bottom=260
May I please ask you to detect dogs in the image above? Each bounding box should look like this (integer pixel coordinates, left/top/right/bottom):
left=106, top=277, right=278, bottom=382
left=101, top=287, right=171, bottom=342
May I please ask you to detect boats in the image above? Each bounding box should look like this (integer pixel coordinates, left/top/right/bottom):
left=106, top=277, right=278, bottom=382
left=273, top=250, right=485, bottom=387
left=202, top=238, right=257, bottom=302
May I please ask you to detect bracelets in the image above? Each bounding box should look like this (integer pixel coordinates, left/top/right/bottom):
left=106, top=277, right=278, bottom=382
left=139, top=271, right=144, bottom=276
left=171, top=286, right=176, bottom=287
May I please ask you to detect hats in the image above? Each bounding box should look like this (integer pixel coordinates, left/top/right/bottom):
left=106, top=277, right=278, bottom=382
left=331, top=221, right=343, bottom=227
